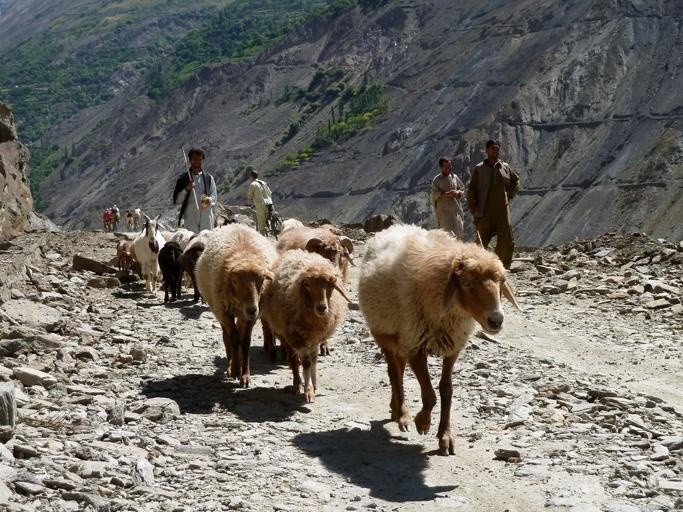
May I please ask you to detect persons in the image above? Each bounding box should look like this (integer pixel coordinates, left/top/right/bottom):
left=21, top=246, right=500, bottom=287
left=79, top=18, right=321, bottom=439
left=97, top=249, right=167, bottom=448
left=245, top=168, right=272, bottom=236
left=110, top=205, right=119, bottom=223
left=464, top=137, right=520, bottom=269
left=172, top=147, right=218, bottom=235
left=430, top=156, right=465, bottom=241
left=132, top=207, right=140, bottom=228
left=124, top=210, right=134, bottom=228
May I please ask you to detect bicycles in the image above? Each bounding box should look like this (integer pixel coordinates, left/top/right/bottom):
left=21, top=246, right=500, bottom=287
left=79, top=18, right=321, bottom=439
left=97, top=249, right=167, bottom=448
left=245, top=204, right=288, bottom=241
left=103, top=217, right=139, bottom=232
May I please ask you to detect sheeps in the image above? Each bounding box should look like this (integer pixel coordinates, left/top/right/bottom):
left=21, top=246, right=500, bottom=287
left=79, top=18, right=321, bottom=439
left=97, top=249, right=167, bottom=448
left=195, top=223, right=277, bottom=390
left=275, top=218, right=355, bottom=357
left=358, top=223, right=523, bottom=456
left=262, top=247, right=352, bottom=406
left=114, top=213, right=212, bottom=307
